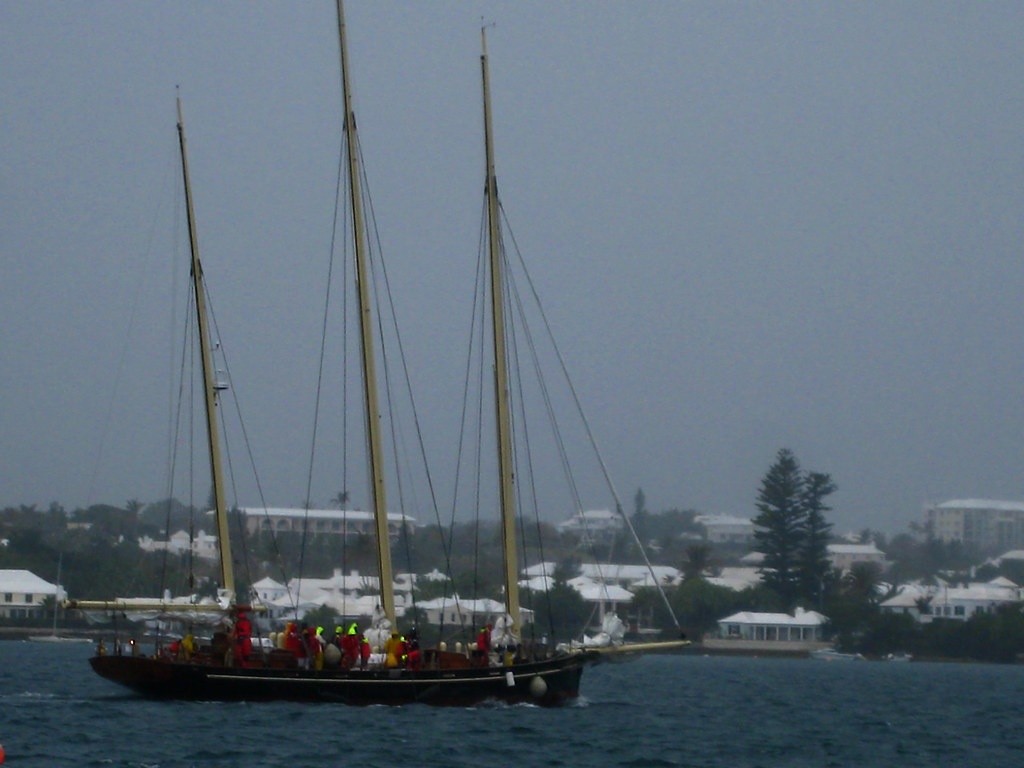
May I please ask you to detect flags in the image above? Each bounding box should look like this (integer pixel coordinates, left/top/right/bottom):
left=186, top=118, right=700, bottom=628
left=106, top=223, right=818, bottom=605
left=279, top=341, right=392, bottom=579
left=482, top=20, right=495, bottom=28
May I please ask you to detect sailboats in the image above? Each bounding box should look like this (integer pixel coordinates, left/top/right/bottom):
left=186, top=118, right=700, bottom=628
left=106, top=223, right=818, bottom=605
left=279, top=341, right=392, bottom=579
left=86, top=0, right=693, bottom=709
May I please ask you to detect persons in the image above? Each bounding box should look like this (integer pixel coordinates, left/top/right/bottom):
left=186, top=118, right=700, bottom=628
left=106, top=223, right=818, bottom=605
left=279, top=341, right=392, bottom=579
left=231, top=614, right=251, bottom=662
left=286, top=621, right=370, bottom=671
left=382, top=626, right=421, bottom=670
left=478, top=624, right=494, bottom=667
left=181, top=634, right=193, bottom=659
